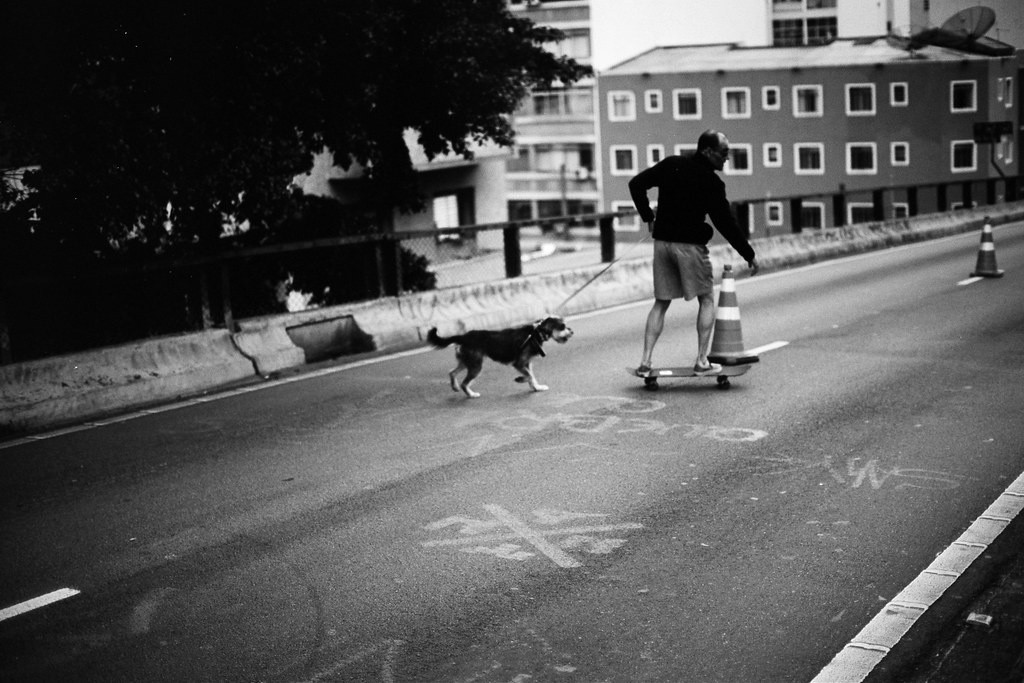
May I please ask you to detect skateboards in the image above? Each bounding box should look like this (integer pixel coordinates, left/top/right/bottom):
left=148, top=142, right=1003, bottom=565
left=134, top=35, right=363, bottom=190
left=624, top=365, right=752, bottom=390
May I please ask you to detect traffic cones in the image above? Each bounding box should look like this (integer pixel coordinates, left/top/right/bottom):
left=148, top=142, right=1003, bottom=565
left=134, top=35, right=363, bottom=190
left=969, top=215, right=1007, bottom=278
left=704, top=263, right=760, bottom=367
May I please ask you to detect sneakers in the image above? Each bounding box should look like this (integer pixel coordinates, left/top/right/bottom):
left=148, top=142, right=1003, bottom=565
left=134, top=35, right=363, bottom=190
left=635, top=365, right=652, bottom=377
left=693, top=360, right=723, bottom=376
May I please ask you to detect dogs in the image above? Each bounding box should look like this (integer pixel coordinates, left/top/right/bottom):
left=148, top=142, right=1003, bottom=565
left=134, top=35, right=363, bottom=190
left=425, top=317, right=574, bottom=396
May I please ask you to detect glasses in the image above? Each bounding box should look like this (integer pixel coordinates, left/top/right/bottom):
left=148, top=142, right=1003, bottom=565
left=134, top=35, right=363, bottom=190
left=716, top=148, right=730, bottom=156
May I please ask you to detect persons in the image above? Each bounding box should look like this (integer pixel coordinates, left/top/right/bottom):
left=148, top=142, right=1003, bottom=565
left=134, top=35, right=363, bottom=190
left=628, top=129, right=759, bottom=376
